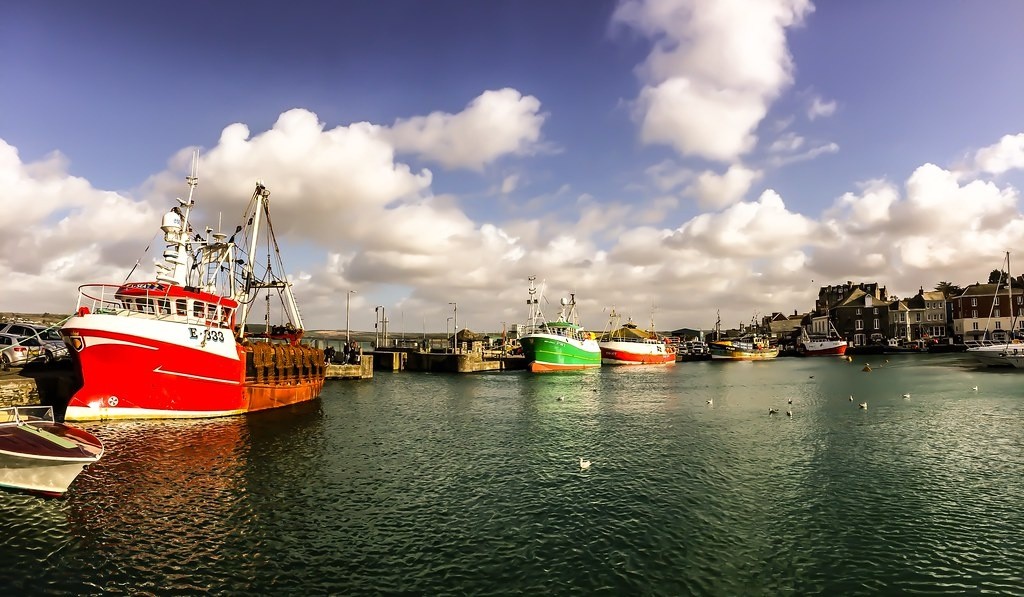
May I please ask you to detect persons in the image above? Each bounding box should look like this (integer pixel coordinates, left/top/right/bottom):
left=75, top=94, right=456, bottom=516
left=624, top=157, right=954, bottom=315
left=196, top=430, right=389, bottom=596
left=324, top=339, right=357, bottom=364
left=423, top=340, right=427, bottom=352
left=234, top=323, right=249, bottom=332
left=758, top=345, right=762, bottom=350
left=582, top=332, right=591, bottom=341
left=511, top=346, right=522, bottom=355
left=272, top=325, right=283, bottom=334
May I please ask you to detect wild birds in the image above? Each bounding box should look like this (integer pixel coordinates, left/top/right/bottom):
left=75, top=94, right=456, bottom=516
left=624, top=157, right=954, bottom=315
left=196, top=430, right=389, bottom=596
left=556, top=395, right=565, bottom=402
left=864, top=363, right=870, bottom=366
left=578, top=455, right=592, bottom=468
left=972, top=385, right=978, bottom=391
left=848, top=395, right=867, bottom=410
left=706, top=398, right=713, bottom=405
left=901, top=393, right=910, bottom=399
left=768, top=398, right=793, bottom=420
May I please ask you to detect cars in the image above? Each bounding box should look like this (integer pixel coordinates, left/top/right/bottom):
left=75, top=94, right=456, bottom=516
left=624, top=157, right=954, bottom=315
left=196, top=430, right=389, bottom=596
left=0, top=332, right=46, bottom=372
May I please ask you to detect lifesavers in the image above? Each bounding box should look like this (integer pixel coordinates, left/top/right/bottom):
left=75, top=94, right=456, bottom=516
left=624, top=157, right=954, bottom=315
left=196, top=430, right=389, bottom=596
left=583, top=333, right=590, bottom=339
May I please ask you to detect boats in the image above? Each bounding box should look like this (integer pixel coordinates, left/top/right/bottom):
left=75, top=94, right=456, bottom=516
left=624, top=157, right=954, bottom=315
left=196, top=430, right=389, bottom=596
left=594, top=303, right=679, bottom=366
left=0, top=404, right=104, bottom=500
left=59, top=146, right=328, bottom=422
left=516, top=274, right=602, bottom=375
left=676, top=326, right=848, bottom=370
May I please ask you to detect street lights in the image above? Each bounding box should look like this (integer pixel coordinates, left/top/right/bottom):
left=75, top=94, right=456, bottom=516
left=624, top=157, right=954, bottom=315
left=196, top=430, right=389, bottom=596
left=447, top=316, right=453, bottom=347
left=347, top=291, right=355, bottom=343
left=449, top=302, right=458, bottom=349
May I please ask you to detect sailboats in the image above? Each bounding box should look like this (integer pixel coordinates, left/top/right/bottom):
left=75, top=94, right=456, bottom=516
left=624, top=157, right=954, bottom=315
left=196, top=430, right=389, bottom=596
left=964, top=250, right=1024, bottom=370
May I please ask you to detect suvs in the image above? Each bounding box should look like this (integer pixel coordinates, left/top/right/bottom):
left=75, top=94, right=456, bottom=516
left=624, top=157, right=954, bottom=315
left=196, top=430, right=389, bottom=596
left=0, top=322, right=75, bottom=364
